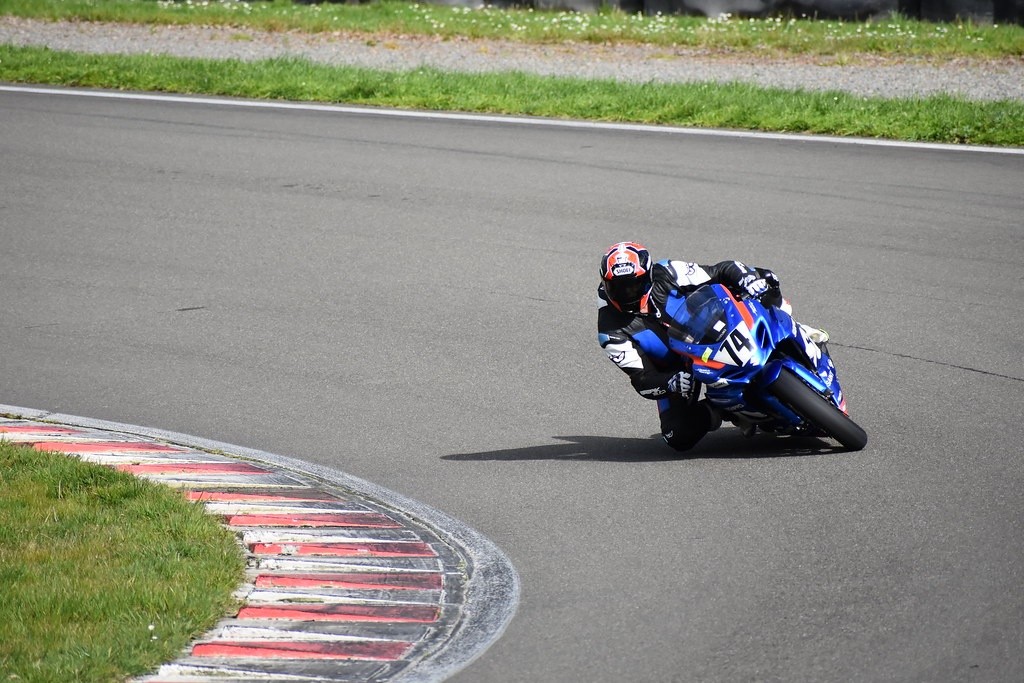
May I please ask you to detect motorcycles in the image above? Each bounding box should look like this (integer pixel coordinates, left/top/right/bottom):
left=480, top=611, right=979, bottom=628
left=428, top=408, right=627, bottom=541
left=666, top=277, right=869, bottom=453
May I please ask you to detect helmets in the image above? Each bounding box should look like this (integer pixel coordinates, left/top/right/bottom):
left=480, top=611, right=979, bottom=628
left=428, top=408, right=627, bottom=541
left=601, top=241, right=654, bottom=314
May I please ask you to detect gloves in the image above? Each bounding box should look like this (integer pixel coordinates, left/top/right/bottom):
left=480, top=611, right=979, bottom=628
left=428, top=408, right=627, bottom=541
left=667, top=372, right=692, bottom=395
left=742, top=275, right=769, bottom=298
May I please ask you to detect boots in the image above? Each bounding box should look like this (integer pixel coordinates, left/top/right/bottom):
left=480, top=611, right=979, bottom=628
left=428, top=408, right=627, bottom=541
left=700, top=396, right=755, bottom=438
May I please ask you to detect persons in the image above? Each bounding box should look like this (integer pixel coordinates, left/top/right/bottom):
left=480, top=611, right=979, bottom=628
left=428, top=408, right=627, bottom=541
left=596, top=242, right=790, bottom=451
